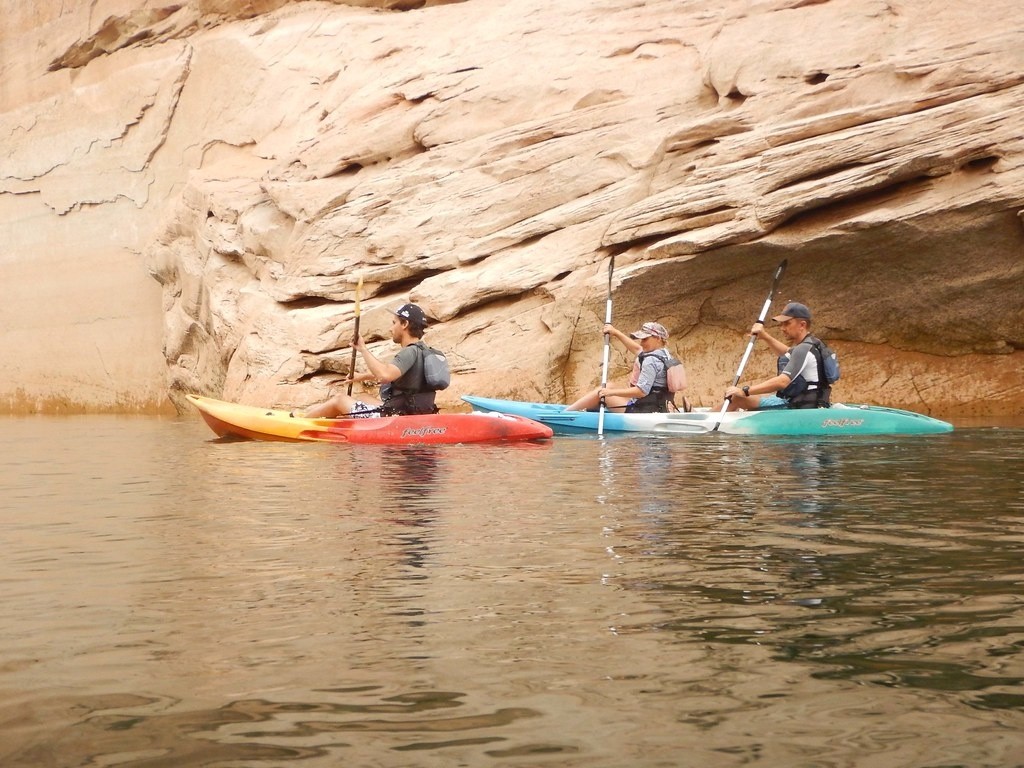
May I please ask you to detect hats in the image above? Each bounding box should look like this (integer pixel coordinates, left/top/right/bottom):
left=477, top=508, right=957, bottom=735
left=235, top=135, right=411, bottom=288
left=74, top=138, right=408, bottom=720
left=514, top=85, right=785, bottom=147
left=772, top=302, right=811, bottom=321
left=630, top=322, right=669, bottom=340
left=385, top=304, right=428, bottom=329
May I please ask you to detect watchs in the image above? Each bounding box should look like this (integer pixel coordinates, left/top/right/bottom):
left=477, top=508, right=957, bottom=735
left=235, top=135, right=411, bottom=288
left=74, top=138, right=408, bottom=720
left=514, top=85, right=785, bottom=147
left=742, top=386, right=750, bottom=396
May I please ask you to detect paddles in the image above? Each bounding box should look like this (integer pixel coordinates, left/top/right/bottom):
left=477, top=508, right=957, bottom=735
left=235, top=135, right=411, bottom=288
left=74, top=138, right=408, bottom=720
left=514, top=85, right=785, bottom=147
left=712, top=258, right=788, bottom=431
left=348, top=276, right=364, bottom=395
left=599, top=253, right=615, bottom=434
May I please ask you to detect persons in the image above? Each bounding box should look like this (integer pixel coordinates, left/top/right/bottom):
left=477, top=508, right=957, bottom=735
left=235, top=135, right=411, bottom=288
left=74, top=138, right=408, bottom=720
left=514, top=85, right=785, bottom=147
left=303, top=303, right=430, bottom=418
left=562, top=322, right=672, bottom=413
left=708, top=303, right=828, bottom=411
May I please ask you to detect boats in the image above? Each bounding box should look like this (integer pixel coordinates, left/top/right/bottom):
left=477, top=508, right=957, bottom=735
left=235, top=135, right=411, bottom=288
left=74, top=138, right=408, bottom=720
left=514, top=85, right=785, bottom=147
left=460, top=395, right=955, bottom=438
left=185, top=390, right=553, bottom=446
